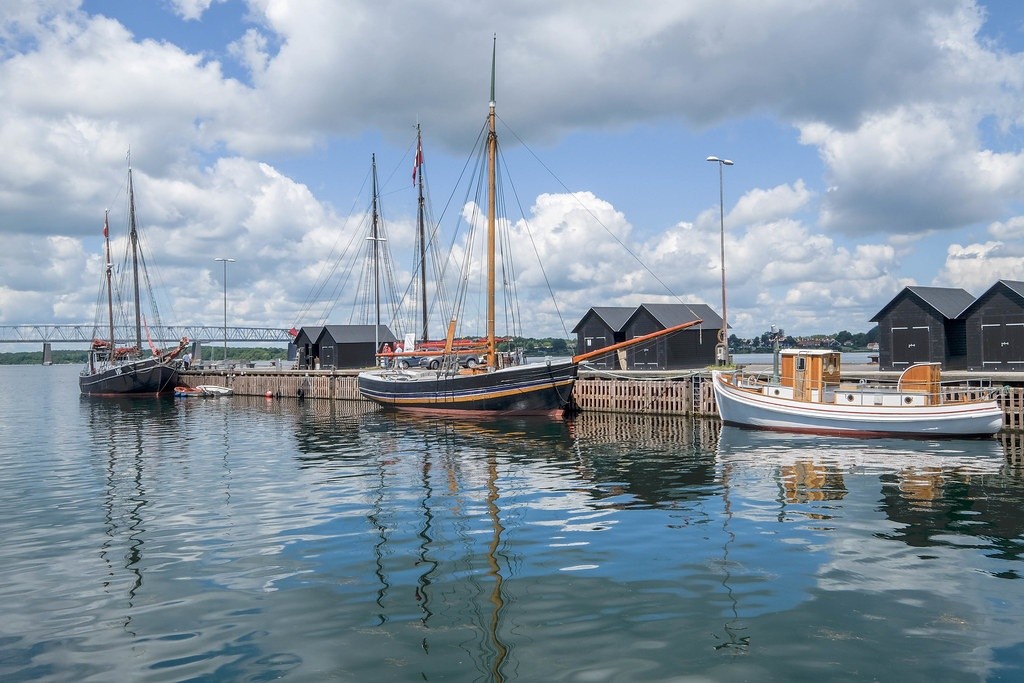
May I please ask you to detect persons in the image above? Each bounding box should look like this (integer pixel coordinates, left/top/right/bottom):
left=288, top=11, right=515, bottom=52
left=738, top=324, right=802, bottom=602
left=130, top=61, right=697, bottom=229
left=393, top=344, right=403, bottom=371
left=188, top=352, right=192, bottom=367
left=183, top=352, right=190, bottom=371
left=382, top=343, right=391, bottom=370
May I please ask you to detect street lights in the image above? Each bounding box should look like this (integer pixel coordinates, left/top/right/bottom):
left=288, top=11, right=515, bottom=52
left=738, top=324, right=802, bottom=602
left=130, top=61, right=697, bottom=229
left=214, top=258, right=236, bottom=369
left=706, top=157, right=734, bottom=368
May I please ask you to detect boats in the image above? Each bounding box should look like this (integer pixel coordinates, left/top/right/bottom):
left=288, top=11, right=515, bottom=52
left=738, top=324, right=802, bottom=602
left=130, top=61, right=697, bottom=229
left=711, top=324, right=1005, bottom=439
left=174, top=386, right=204, bottom=397
left=712, top=424, right=1004, bottom=522
left=196, top=385, right=233, bottom=396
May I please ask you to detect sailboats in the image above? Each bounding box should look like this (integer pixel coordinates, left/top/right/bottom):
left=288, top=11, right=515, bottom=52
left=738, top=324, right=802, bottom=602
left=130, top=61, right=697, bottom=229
left=78, top=142, right=190, bottom=398
left=293, top=32, right=705, bottom=418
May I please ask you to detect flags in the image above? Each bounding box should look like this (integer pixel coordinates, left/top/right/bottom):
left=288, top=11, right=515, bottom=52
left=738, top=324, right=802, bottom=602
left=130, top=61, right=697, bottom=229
left=103, top=215, right=108, bottom=238
left=412, top=137, right=424, bottom=188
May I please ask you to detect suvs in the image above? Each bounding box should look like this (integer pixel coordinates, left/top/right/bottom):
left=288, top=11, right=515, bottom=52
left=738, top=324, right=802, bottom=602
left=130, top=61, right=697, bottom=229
left=419, top=347, right=479, bottom=369
left=395, top=346, right=445, bottom=369
